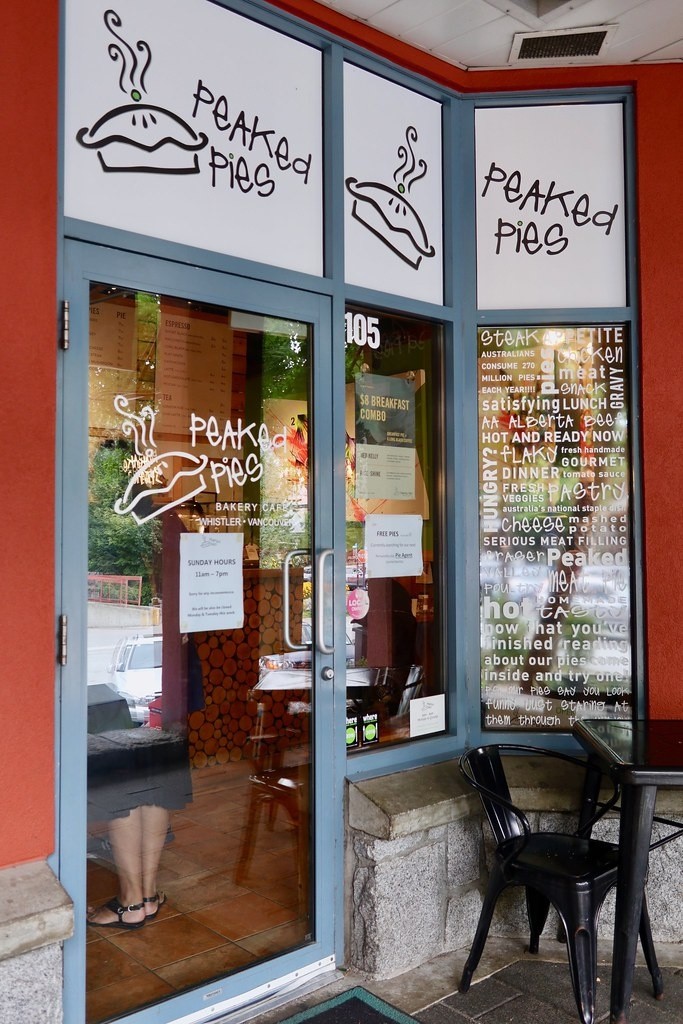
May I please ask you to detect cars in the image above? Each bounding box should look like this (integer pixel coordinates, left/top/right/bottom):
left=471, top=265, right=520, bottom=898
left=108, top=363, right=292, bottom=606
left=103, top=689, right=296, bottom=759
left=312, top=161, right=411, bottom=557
left=302, top=563, right=366, bottom=590
left=108, top=633, right=163, bottom=724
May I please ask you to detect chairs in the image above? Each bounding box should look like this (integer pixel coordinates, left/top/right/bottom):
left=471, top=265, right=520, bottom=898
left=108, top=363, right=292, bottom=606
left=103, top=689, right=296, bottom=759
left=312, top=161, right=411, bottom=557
left=457, top=743, right=667, bottom=1024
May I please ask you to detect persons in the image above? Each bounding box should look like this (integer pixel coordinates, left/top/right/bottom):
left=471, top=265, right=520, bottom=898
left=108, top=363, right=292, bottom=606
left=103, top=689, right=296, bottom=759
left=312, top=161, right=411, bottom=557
left=87, top=438, right=204, bottom=927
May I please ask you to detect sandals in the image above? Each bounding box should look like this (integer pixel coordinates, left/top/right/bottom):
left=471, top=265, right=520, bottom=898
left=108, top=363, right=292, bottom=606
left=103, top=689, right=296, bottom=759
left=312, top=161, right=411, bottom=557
left=142, top=890, right=167, bottom=920
left=86, top=896, right=146, bottom=930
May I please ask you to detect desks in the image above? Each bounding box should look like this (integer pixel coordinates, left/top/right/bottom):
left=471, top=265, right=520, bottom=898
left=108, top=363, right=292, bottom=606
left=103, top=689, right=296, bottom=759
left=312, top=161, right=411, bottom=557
left=231, top=666, right=395, bottom=933
left=557, top=720, right=683, bottom=1024
